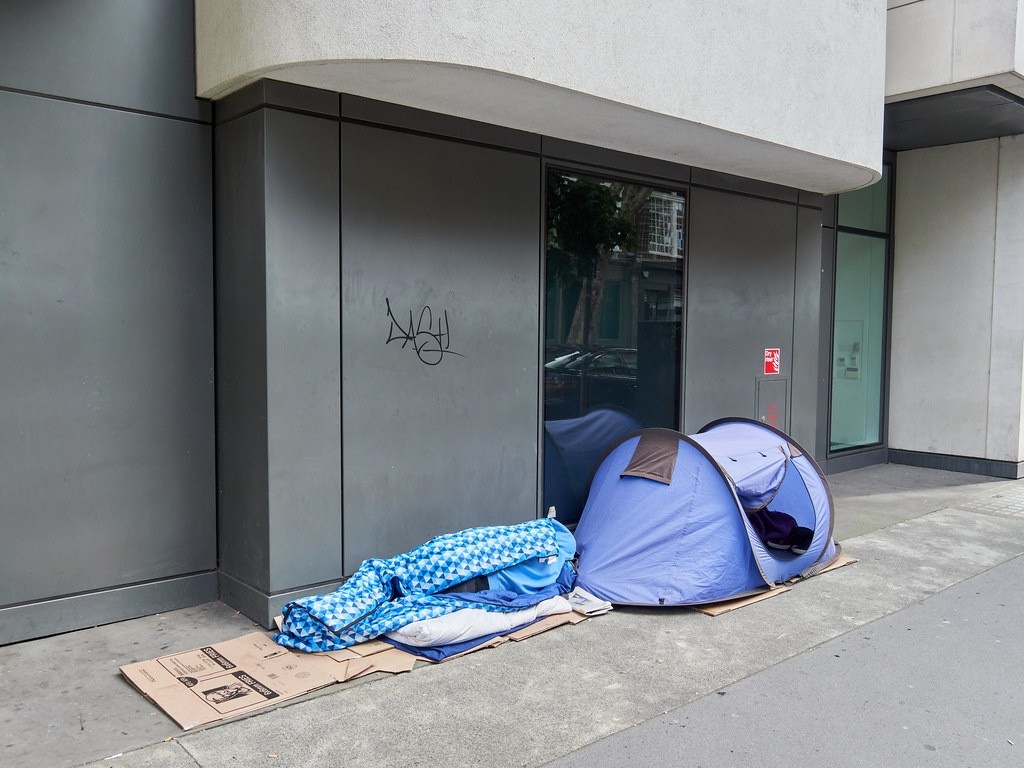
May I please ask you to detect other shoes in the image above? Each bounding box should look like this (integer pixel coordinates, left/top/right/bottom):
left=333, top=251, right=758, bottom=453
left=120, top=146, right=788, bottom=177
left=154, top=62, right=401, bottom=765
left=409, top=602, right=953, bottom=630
left=768, top=526, right=814, bottom=554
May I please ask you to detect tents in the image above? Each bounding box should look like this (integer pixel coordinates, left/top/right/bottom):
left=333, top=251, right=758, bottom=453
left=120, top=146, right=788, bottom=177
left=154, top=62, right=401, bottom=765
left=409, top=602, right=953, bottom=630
left=543, top=403, right=643, bottom=526
left=572, top=417, right=842, bottom=606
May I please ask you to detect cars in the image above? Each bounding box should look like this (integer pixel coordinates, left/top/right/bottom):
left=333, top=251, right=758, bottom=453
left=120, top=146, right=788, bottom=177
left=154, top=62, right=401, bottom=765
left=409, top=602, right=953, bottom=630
left=544, top=345, right=638, bottom=405
